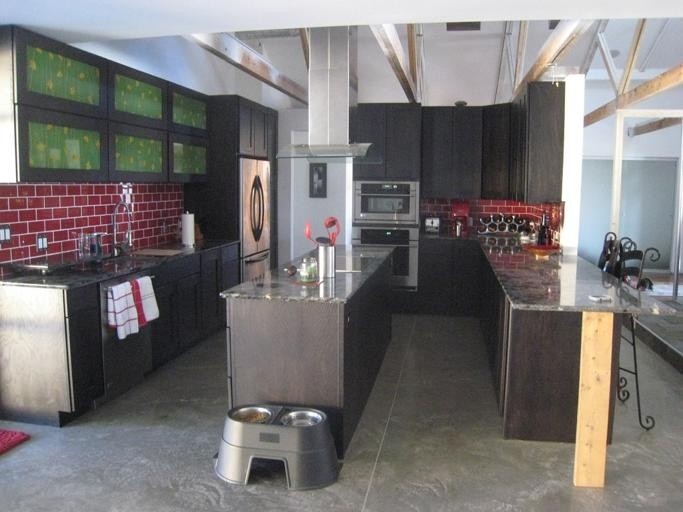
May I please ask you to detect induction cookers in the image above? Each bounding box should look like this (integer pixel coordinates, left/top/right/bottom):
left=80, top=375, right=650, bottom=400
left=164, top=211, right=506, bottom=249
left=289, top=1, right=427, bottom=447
left=332, top=254, right=376, bottom=276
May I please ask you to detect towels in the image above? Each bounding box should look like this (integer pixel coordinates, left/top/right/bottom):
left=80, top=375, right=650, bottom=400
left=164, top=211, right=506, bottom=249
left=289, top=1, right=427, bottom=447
left=135, top=274, right=160, bottom=321
left=106, top=281, right=139, bottom=342
left=128, top=279, right=147, bottom=328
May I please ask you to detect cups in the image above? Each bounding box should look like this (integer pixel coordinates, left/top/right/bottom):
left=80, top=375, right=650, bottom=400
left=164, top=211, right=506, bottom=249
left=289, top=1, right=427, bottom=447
left=319, top=278, right=335, bottom=299
left=316, top=243, right=337, bottom=280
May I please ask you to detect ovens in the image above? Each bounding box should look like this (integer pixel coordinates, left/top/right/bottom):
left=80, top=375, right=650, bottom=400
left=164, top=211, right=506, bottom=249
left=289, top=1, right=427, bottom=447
left=98, top=273, right=152, bottom=398
left=352, top=176, right=418, bottom=292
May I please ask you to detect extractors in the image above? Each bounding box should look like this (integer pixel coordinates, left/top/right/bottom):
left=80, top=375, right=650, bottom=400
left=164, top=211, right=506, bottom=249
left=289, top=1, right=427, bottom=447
left=268, top=24, right=376, bottom=165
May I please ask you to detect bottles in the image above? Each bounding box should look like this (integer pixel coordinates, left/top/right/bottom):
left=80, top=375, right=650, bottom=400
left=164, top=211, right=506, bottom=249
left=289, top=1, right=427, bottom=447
left=482, top=238, right=528, bottom=257
left=478, top=213, right=532, bottom=234
left=538, top=209, right=550, bottom=247
left=456, top=221, right=462, bottom=237
left=71, top=232, right=103, bottom=268
left=299, top=256, right=320, bottom=283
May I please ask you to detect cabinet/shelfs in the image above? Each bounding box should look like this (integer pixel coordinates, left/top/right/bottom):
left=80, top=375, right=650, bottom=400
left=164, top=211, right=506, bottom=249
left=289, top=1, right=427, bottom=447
left=421, top=233, right=479, bottom=317
left=0, top=282, right=105, bottom=427
left=150, top=250, right=199, bottom=372
left=0, top=20, right=210, bottom=187
left=352, top=102, right=422, bottom=182
left=237, top=251, right=272, bottom=282
left=483, top=103, right=512, bottom=203
left=479, top=232, right=678, bottom=445
left=205, top=94, right=276, bottom=159
left=199, top=239, right=241, bottom=341
left=513, top=81, right=565, bottom=206
left=423, top=105, right=482, bottom=202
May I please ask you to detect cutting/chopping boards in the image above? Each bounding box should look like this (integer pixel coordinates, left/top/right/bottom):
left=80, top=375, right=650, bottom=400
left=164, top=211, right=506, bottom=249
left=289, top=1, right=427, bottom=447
left=132, top=247, right=184, bottom=257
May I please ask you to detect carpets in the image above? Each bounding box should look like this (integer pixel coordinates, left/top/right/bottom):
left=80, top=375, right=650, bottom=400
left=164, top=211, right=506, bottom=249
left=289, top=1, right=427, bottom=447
left=0, top=426, right=31, bottom=455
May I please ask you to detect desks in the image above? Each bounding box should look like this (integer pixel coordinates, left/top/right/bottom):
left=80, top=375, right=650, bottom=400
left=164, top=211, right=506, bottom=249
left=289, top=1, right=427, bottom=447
left=217, top=241, right=397, bottom=459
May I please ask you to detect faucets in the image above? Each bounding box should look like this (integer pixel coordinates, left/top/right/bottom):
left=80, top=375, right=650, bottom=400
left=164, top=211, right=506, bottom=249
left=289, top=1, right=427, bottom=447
left=111, top=201, right=133, bottom=258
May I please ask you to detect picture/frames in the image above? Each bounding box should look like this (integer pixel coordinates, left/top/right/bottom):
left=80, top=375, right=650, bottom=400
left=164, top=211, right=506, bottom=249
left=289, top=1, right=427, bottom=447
left=307, top=162, right=328, bottom=198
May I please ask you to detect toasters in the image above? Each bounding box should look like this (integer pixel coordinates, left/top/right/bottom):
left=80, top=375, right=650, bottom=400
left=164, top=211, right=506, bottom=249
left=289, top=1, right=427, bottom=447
left=423, top=217, right=445, bottom=235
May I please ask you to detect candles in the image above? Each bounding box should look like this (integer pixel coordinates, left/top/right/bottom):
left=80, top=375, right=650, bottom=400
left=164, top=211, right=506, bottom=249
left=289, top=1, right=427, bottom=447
left=179, top=209, right=195, bottom=248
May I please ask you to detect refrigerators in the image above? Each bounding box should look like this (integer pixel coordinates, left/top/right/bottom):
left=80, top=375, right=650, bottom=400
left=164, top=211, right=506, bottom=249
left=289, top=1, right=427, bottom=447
left=237, top=155, right=272, bottom=285
